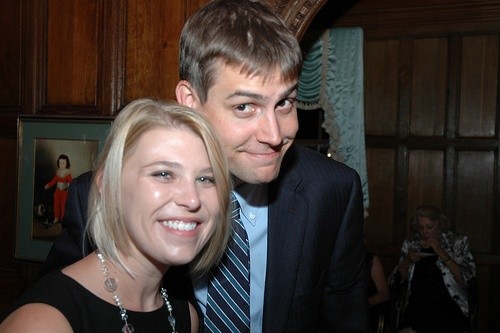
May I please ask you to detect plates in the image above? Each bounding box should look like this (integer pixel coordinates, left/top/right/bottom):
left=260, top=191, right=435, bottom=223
left=414, top=253, right=434, bottom=256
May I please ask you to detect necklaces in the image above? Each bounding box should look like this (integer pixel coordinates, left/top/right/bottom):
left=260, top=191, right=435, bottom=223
left=95, top=250, right=179, bottom=333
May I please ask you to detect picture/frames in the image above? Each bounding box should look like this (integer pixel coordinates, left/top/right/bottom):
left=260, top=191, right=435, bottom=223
left=12, top=114, right=116, bottom=265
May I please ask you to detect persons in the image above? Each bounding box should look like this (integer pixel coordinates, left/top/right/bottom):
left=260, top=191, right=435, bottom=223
left=387, top=203, right=477, bottom=333
left=173, top=1, right=376, bottom=333
left=0, top=97, right=235, bottom=333
left=366, top=240, right=389, bottom=308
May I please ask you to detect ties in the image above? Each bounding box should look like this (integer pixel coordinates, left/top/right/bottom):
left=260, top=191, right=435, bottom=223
left=203, top=190, right=254, bottom=333
